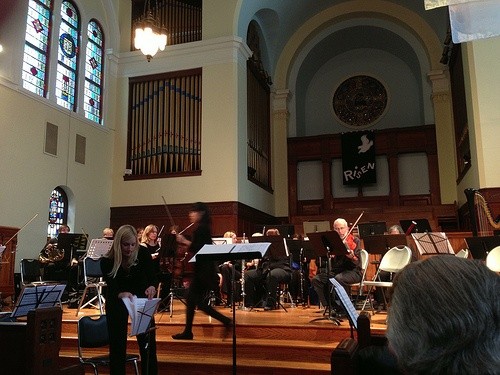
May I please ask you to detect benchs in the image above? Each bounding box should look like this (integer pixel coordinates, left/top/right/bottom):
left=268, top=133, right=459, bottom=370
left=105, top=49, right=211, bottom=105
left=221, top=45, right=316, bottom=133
left=0, top=306, right=85, bottom=375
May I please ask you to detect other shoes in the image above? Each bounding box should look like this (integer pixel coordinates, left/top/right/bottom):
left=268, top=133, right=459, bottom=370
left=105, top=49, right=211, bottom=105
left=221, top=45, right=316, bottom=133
left=222, top=319, right=236, bottom=330
left=263, top=303, right=277, bottom=310
left=170, top=331, right=194, bottom=340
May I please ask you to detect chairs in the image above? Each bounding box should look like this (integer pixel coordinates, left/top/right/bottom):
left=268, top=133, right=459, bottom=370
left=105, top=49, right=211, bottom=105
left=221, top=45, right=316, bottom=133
left=20, top=240, right=500, bottom=375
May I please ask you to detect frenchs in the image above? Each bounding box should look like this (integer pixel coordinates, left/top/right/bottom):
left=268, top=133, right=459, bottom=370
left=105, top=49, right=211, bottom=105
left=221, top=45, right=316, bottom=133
left=39, top=242, right=65, bottom=265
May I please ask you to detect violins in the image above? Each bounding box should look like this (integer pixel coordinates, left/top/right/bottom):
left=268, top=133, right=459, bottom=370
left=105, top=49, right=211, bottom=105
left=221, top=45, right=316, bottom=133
left=342, top=233, right=360, bottom=265
left=173, top=225, right=194, bottom=279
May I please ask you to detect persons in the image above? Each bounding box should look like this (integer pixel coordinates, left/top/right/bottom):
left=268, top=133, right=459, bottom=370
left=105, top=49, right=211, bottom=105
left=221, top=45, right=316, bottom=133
left=373, top=223, right=412, bottom=310
left=311, top=213, right=362, bottom=317
left=100, top=223, right=159, bottom=375
left=172, top=202, right=237, bottom=341
left=37, top=225, right=309, bottom=311
left=386, top=253, right=500, bottom=375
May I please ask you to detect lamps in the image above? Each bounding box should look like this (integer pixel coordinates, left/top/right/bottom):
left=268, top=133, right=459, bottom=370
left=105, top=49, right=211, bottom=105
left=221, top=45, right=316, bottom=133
left=133, top=0, right=169, bottom=63
